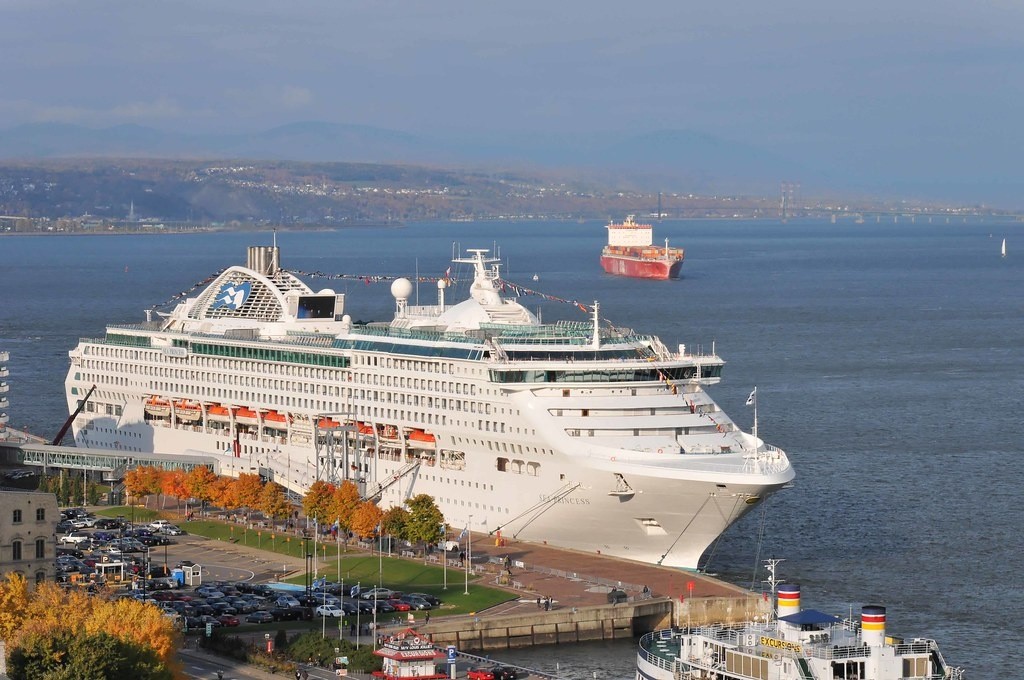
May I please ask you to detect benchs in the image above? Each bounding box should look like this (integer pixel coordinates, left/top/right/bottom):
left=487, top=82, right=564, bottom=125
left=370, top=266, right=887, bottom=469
left=357, top=540, right=372, bottom=549
left=512, top=579, right=525, bottom=590
left=294, top=529, right=309, bottom=537
left=476, top=563, right=488, bottom=572
left=448, top=559, right=463, bottom=568
left=402, top=550, right=418, bottom=558
left=428, top=555, right=440, bottom=563
left=336, top=536, right=349, bottom=544
left=217, top=514, right=229, bottom=520
left=236, top=517, right=248, bottom=524
left=318, top=533, right=330, bottom=541
left=200, top=510, right=212, bottom=517
left=275, top=524, right=287, bottom=532
left=257, top=522, right=269, bottom=528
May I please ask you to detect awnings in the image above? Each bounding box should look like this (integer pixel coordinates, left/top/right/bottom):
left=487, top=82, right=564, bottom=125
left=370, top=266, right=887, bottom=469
left=778, top=608, right=842, bottom=625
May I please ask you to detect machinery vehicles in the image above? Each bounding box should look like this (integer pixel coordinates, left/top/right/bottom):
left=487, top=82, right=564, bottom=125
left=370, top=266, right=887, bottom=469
left=44, top=385, right=98, bottom=446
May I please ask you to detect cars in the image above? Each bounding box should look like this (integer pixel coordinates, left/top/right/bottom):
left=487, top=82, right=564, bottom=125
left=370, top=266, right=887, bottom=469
left=56, top=506, right=440, bottom=629
left=5, top=469, right=34, bottom=480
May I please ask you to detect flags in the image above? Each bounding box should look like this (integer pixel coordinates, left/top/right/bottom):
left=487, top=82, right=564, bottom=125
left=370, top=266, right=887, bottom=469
left=407, top=613, right=415, bottom=623
left=446, top=267, right=451, bottom=276
left=312, top=518, right=316, bottom=524
left=746, top=390, right=755, bottom=405
left=331, top=518, right=338, bottom=531
left=373, top=525, right=380, bottom=533
left=440, top=524, right=445, bottom=533
left=455, top=527, right=467, bottom=542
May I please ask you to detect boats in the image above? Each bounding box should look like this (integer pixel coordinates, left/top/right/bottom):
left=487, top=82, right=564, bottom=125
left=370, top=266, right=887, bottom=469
left=636, top=557, right=966, bottom=680
left=600, top=212, right=685, bottom=280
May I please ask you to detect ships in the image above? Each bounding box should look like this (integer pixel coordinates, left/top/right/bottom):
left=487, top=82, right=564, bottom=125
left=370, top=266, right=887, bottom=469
left=64, top=227, right=797, bottom=573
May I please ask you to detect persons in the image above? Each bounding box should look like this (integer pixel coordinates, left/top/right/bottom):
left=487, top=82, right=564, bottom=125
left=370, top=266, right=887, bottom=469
left=350, top=610, right=430, bottom=636
left=497, top=527, right=503, bottom=549
left=425, top=540, right=438, bottom=556
left=225, top=511, right=248, bottom=526
left=217, top=668, right=224, bottom=680
left=536, top=595, right=553, bottom=611
left=294, top=658, right=320, bottom=680
left=185, top=510, right=194, bottom=522
left=458, top=547, right=470, bottom=562
left=282, top=515, right=374, bottom=544
left=503, top=555, right=512, bottom=576
left=611, top=585, right=648, bottom=606
